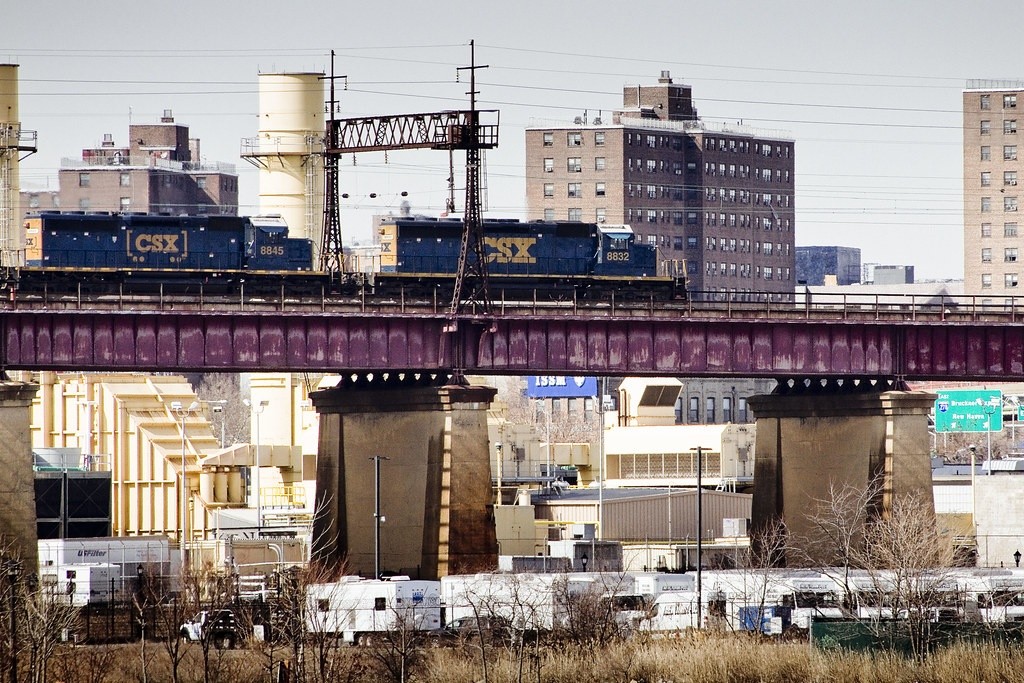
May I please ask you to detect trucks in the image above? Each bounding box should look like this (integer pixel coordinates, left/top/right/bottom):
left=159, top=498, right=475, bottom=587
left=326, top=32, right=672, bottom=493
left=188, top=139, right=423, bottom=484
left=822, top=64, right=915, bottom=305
left=441, top=568, right=1024, bottom=650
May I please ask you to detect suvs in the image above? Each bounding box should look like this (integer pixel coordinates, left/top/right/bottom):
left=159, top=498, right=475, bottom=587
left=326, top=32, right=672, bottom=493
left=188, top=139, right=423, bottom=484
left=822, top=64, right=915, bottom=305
left=178, top=609, right=237, bottom=645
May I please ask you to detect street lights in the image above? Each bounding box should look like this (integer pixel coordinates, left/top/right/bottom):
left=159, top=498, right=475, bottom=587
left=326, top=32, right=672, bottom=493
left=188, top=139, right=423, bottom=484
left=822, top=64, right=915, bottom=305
left=969, top=445, right=977, bottom=530
left=243, top=398, right=269, bottom=539
left=365, top=452, right=390, bottom=577
left=690, top=446, right=713, bottom=630
left=977, top=397, right=1001, bottom=475
left=171, top=401, right=198, bottom=575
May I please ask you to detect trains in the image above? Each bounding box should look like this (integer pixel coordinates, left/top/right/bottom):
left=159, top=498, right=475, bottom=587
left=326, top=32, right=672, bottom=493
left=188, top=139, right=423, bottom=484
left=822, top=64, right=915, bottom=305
left=0, top=210, right=689, bottom=302
